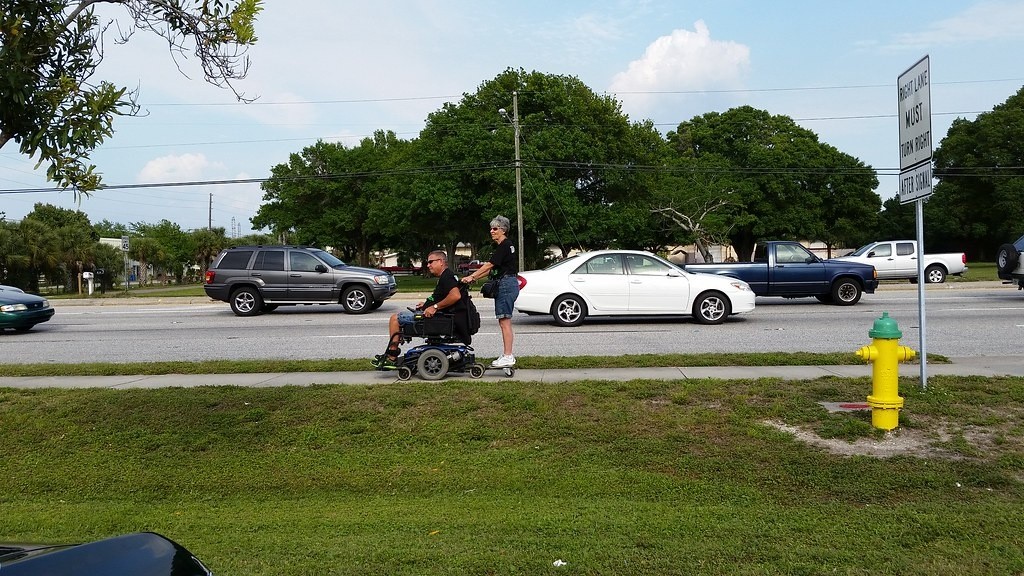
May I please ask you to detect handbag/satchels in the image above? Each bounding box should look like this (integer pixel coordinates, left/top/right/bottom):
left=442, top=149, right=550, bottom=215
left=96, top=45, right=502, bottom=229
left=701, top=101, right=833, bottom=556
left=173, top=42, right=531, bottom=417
left=480, top=281, right=498, bottom=298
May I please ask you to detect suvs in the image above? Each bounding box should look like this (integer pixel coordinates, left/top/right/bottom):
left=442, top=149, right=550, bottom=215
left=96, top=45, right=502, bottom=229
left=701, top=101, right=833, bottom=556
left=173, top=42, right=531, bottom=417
left=203, top=244, right=399, bottom=318
left=996, top=233, right=1024, bottom=294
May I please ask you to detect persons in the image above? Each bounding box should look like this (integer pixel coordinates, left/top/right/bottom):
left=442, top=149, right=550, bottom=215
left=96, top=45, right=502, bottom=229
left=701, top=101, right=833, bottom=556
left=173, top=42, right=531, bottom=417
left=629, top=258, right=657, bottom=273
left=371, top=251, right=460, bottom=368
left=460, top=215, right=519, bottom=367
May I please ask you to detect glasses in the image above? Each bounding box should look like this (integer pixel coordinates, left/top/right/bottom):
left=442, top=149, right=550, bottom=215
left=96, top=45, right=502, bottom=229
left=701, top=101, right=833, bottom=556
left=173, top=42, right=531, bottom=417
left=427, top=259, right=439, bottom=264
left=491, top=227, right=502, bottom=231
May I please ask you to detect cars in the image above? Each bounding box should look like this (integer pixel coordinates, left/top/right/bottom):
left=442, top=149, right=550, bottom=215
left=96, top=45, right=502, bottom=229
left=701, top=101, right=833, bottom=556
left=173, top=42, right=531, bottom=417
left=514, top=247, right=756, bottom=329
left=0, top=527, right=219, bottom=576
left=0, top=281, right=55, bottom=334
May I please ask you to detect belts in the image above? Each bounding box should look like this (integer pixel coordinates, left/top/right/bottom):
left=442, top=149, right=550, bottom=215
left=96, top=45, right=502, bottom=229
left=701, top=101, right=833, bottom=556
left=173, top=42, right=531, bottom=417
left=494, top=273, right=516, bottom=280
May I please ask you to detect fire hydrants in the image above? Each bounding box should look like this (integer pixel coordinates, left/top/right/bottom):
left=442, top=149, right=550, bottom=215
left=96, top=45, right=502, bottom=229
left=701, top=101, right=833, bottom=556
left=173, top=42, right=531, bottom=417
left=854, top=311, right=917, bottom=432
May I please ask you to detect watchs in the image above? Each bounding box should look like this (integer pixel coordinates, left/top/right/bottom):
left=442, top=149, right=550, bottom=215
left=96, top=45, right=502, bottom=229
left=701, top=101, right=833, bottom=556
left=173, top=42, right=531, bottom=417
left=434, top=304, right=439, bottom=310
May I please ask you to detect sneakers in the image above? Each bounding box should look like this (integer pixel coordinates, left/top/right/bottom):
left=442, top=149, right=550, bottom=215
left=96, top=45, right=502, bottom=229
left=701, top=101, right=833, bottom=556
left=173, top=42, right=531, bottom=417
left=374, top=355, right=385, bottom=360
left=370, top=356, right=398, bottom=368
left=492, top=354, right=516, bottom=366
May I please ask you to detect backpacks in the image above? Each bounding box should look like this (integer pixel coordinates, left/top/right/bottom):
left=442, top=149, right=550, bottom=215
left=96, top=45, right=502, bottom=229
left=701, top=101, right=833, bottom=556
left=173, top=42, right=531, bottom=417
left=464, top=290, right=481, bottom=335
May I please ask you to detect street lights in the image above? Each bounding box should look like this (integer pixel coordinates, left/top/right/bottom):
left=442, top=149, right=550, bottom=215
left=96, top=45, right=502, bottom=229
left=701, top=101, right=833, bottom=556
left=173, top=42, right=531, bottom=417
left=498, top=108, right=527, bottom=272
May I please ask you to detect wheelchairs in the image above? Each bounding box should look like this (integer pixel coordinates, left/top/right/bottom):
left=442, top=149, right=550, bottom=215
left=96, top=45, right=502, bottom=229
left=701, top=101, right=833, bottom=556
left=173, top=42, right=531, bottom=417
left=374, top=280, right=518, bottom=382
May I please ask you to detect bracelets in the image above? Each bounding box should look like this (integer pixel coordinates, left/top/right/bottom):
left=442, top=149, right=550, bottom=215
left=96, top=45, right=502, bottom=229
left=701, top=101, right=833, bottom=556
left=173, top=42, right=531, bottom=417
left=471, top=274, right=475, bottom=280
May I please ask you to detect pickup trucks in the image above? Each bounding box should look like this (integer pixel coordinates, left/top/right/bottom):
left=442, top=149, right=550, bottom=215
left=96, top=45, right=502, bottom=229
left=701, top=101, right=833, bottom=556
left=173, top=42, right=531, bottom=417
left=458, top=259, right=489, bottom=277
left=681, top=240, right=880, bottom=307
left=830, top=240, right=970, bottom=285
